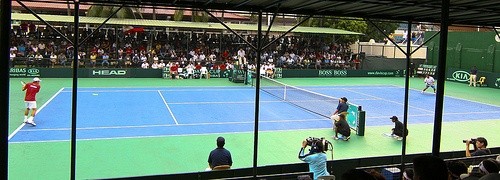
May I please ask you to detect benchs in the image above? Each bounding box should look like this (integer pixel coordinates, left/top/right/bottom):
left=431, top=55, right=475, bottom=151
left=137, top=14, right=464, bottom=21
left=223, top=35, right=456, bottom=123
left=9, top=27, right=343, bottom=69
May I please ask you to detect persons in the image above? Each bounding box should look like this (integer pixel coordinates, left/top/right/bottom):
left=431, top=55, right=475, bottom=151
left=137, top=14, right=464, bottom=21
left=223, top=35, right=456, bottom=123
left=424, top=74, right=435, bottom=91
left=341, top=138, right=500, bottom=180
left=335, top=114, right=351, bottom=141
left=10, top=21, right=425, bottom=79
left=470, top=64, right=477, bottom=88
left=208, top=137, right=232, bottom=170
left=22, top=77, right=41, bottom=126
left=299, top=147, right=329, bottom=178
left=335, top=97, right=349, bottom=114
left=390, top=116, right=408, bottom=137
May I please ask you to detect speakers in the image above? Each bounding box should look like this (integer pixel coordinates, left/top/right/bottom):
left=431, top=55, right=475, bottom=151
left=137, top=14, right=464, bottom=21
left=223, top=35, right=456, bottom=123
left=360, top=52, right=365, bottom=59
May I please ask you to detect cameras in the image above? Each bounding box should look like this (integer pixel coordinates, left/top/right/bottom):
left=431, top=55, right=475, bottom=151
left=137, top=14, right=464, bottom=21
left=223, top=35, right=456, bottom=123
left=463, top=138, right=476, bottom=144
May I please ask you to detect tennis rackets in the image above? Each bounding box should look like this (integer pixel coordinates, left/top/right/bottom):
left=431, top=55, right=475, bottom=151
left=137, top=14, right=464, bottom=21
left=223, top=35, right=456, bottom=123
left=20, top=80, right=26, bottom=85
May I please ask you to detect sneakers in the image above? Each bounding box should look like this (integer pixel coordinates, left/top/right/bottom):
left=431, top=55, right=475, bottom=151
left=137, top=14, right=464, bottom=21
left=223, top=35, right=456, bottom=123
left=343, top=136, right=350, bottom=141
left=330, top=135, right=339, bottom=140
left=25, top=119, right=36, bottom=126
left=397, top=137, right=403, bottom=140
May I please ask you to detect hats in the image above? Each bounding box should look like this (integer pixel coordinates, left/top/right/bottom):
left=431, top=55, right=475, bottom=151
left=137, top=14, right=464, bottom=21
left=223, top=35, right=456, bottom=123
left=217, top=137, right=225, bottom=143
left=34, top=78, right=41, bottom=81
left=390, top=116, right=397, bottom=119
left=341, top=97, right=347, bottom=101
left=338, top=114, right=345, bottom=117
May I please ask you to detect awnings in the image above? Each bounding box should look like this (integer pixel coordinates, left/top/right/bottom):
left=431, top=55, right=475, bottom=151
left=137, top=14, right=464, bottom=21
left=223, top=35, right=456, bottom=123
left=11, top=0, right=499, bottom=37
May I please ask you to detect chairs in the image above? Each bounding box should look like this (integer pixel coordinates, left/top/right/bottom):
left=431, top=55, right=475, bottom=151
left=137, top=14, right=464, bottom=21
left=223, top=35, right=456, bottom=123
left=188, top=69, right=195, bottom=78
left=200, top=67, right=209, bottom=79
left=213, top=165, right=230, bottom=170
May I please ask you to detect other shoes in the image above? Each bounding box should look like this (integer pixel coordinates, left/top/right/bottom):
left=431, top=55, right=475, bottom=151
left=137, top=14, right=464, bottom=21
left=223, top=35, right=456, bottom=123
left=474, top=85, right=476, bottom=87
left=422, top=90, right=424, bottom=93
left=469, top=85, right=472, bottom=86
left=434, top=91, right=436, bottom=93
